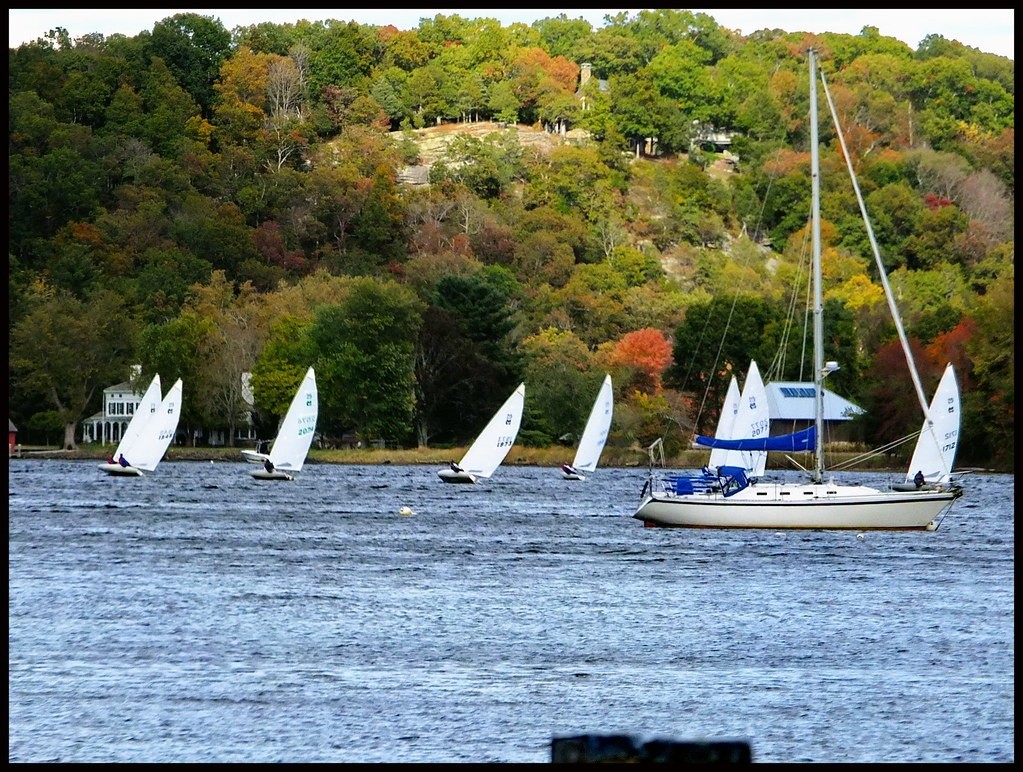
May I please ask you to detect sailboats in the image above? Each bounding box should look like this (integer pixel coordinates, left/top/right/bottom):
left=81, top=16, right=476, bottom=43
left=97, top=372, right=183, bottom=476
left=888, top=362, right=962, bottom=492
left=562, top=374, right=615, bottom=481
left=437, top=382, right=525, bottom=483
left=633, top=45, right=964, bottom=533
left=659, top=375, right=742, bottom=485
left=248, top=365, right=319, bottom=480
left=666, top=359, right=771, bottom=494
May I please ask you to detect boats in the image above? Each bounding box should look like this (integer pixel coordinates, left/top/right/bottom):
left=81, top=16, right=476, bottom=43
left=241, top=449, right=270, bottom=462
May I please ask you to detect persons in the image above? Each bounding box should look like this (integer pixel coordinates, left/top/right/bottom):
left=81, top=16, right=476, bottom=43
left=107, top=454, right=117, bottom=464
left=562, top=461, right=577, bottom=475
left=450, top=460, right=464, bottom=473
left=914, top=471, right=926, bottom=488
left=118, top=453, right=130, bottom=467
left=264, top=459, right=275, bottom=474
